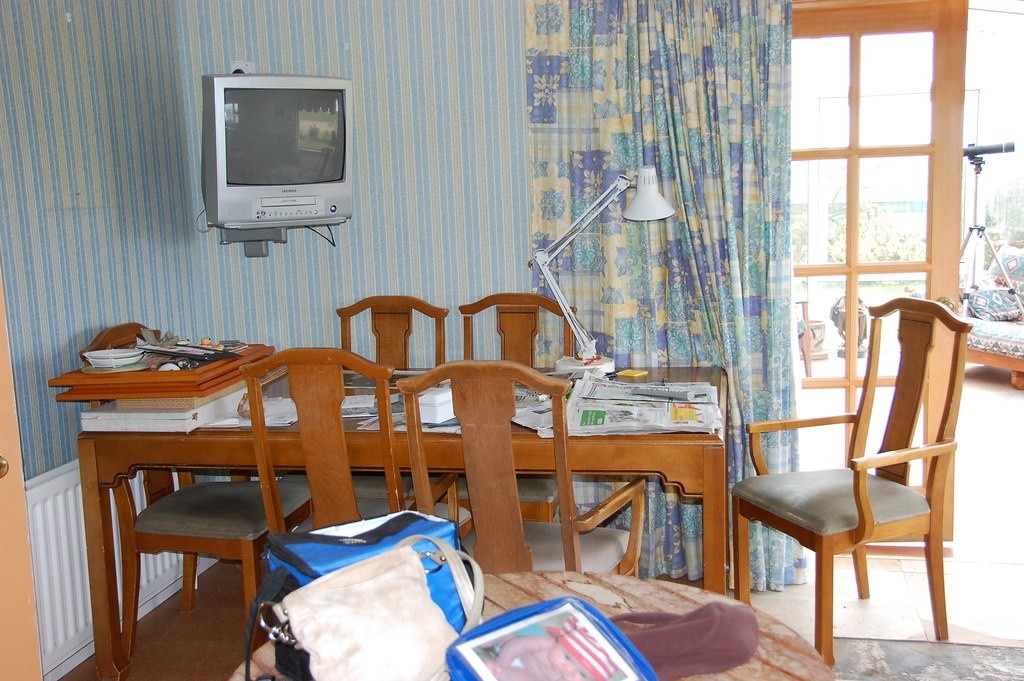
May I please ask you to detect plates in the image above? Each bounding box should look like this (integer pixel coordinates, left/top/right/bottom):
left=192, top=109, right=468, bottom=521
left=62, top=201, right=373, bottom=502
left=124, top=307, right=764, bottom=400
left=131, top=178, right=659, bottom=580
left=82, top=349, right=150, bottom=375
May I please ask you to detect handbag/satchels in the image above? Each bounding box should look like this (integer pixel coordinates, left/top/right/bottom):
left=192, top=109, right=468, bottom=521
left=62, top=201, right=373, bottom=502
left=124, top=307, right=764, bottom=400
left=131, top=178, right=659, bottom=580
left=246, top=508, right=484, bottom=681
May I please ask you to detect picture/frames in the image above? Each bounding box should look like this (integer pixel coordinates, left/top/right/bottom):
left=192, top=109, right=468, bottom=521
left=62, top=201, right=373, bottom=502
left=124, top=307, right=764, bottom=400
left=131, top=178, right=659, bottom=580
left=48, top=344, right=277, bottom=402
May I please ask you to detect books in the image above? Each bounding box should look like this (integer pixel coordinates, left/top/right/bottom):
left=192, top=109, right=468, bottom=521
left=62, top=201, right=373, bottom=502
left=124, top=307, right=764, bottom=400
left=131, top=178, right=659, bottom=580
left=238, top=396, right=299, bottom=427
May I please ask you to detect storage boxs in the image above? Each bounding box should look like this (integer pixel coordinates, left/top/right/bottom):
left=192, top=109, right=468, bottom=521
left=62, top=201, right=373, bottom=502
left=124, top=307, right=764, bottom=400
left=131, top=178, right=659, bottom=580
left=82, top=386, right=249, bottom=433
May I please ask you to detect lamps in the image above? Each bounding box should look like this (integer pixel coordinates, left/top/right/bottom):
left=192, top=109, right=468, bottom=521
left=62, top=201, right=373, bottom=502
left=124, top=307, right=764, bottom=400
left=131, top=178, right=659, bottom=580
left=535, top=166, right=676, bottom=376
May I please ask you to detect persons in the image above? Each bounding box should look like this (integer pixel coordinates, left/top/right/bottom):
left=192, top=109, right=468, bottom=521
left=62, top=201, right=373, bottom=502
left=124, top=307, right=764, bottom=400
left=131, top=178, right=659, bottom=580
left=484, top=616, right=618, bottom=680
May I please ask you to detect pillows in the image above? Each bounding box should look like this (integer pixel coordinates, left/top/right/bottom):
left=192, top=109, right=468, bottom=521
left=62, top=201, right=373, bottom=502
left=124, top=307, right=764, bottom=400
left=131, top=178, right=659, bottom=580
left=986, top=242, right=1024, bottom=295
left=967, top=285, right=1022, bottom=319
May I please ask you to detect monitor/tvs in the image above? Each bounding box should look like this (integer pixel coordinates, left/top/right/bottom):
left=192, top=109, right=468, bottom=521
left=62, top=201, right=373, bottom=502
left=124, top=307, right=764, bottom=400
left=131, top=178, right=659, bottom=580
left=201, top=74, right=354, bottom=225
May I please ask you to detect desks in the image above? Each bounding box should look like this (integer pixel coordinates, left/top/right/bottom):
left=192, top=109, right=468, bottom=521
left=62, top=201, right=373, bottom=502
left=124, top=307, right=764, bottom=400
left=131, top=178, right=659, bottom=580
left=76, top=363, right=730, bottom=677
left=231, top=569, right=835, bottom=681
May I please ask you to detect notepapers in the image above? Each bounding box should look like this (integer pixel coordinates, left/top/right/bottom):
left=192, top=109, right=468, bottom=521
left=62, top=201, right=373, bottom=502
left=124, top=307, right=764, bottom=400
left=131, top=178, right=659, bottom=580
left=616, top=369, right=649, bottom=377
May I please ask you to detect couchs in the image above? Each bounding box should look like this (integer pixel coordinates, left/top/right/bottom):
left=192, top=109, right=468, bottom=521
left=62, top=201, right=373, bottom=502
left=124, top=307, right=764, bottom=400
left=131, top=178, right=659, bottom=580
left=961, top=283, right=1023, bottom=388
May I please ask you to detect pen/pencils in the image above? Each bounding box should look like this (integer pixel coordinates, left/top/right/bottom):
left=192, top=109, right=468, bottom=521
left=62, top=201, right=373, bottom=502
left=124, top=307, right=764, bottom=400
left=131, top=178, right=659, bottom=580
left=662, top=377, right=669, bottom=386
left=605, top=371, right=619, bottom=378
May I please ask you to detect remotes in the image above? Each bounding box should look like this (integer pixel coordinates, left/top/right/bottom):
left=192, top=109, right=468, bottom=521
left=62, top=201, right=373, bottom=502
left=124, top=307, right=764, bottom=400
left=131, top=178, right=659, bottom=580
left=631, top=387, right=694, bottom=401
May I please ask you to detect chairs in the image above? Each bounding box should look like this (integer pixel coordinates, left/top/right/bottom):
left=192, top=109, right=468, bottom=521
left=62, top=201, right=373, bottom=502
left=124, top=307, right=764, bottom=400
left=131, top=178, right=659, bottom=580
left=92, top=290, right=727, bottom=668
left=733, top=296, right=972, bottom=667
